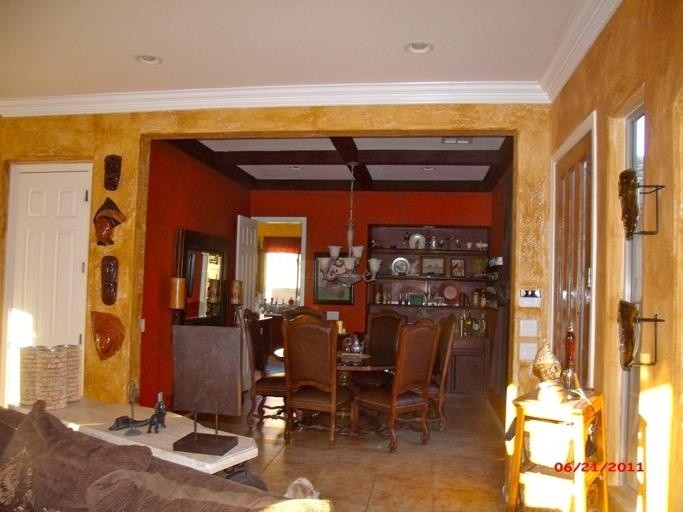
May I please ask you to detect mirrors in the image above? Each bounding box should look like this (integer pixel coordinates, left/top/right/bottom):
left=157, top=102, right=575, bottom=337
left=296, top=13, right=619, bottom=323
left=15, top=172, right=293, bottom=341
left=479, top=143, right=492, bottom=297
left=172, top=229, right=231, bottom=327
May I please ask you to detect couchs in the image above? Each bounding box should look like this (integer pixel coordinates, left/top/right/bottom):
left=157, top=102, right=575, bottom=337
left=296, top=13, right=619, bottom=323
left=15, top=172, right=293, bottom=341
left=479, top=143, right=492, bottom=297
left=2, top=403, right=331, bottom=511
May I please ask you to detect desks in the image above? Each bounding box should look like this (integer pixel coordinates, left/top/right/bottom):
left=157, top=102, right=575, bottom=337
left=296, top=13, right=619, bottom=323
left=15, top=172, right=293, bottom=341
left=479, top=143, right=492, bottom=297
left=5, top=393, right=269, bottom=491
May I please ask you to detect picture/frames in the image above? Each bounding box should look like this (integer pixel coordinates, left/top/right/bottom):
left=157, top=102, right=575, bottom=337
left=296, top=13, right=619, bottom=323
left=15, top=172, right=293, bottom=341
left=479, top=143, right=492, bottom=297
left=450, top=257, right=466, bottom=278
left=418, top=254, right=446, bottom=277
left=312, top=251, right=355, bottom=306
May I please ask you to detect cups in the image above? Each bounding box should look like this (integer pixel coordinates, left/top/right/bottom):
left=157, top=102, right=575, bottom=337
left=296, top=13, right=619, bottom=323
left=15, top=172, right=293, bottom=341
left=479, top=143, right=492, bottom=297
left=410, top=294, right=423, bottom=305
left=398, top=293, right=408, bottom=305
left=467, top=242, right=471, bottom=249
left=336, top=320, right=343, bottom=334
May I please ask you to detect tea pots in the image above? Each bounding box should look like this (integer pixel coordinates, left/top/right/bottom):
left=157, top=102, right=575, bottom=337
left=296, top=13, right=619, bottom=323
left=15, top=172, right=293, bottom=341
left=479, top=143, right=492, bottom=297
left=344, top=335, right=365, bottom=354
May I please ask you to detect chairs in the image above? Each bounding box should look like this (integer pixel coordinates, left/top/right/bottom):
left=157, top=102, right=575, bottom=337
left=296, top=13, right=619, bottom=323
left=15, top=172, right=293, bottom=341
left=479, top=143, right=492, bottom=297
left=238, top=307, right=458, bottom=450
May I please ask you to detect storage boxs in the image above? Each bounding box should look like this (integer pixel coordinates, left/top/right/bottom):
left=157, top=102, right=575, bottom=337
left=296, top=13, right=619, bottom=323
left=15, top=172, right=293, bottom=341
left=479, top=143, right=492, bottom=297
left=520, top=470, right=592, bottom=511
left=522, top=416, right=591, bottom=471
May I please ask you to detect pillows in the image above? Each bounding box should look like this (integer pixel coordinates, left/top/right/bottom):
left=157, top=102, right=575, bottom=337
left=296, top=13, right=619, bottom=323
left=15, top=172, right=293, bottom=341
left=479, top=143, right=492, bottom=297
left=2, top=391, right=153, bottom=510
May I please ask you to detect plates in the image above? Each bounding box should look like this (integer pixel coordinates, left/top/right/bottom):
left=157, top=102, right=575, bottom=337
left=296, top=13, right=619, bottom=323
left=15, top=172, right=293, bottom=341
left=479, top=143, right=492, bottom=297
left=438, top=280, right=461, bottom=305
left=392, top=257, right=411, bottom=277
left=409, top=234, right=425, bottom=249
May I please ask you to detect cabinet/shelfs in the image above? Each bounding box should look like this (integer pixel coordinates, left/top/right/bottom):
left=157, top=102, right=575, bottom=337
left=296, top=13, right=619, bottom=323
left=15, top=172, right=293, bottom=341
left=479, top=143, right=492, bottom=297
left=368, top=223, right=492, bottom=393
left=507, top=389, right=609, bottom=510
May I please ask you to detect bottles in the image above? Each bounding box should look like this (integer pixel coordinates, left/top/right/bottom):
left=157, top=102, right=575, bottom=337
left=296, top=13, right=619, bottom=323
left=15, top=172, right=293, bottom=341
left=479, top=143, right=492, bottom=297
left=403, top=231, right=409, bottom=249
left=562, top=322, right=575, bottom=388
left=260, top=297, right=296, bottom=314
left=373, top=285, right=391, bottom=305
left=459, top=288, right=486, bottom=308
left=457, top=312, right=486, bottom=338
left=532, top=339, right=561, bottom=383
left=426, top=234, right=437, bottom=250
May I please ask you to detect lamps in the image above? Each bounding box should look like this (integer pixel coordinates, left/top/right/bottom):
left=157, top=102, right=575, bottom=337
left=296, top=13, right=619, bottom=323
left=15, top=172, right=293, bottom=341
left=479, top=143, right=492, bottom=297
left=230, top=279, right=245, bottom=325
left=170, top=276, right=188, bottom=325
left=205, top=273, right=219, bottom=319
left=316, top=162, right=381, bottom=287
